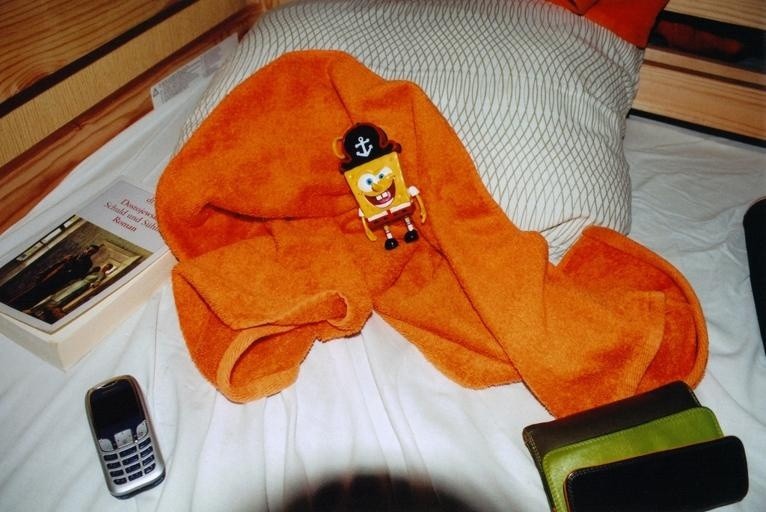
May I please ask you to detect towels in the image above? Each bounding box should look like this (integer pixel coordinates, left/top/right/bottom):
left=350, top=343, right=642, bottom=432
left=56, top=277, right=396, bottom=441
left=152, top=47, right=712, bottom=421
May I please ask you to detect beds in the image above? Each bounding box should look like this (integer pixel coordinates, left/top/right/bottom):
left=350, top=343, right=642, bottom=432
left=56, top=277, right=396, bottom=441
left=1, top=0, right=766, bottom=512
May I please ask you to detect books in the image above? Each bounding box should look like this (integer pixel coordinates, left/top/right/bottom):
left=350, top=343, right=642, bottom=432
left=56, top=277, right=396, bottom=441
left=0, top=175, right=180, bottom=372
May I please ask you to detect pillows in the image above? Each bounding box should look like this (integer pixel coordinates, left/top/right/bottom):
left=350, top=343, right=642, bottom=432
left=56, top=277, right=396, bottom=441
left=178, top=1, right=664, bottom=263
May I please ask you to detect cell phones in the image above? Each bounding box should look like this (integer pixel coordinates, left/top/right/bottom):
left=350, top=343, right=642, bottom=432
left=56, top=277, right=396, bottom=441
left=84, top=374, right=166, bottom=499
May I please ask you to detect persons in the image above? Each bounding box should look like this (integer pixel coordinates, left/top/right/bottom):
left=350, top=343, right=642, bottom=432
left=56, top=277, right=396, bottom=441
left=8, top=245, right=99, bottom=311
left=34, top=262, right=113, bottom=320
